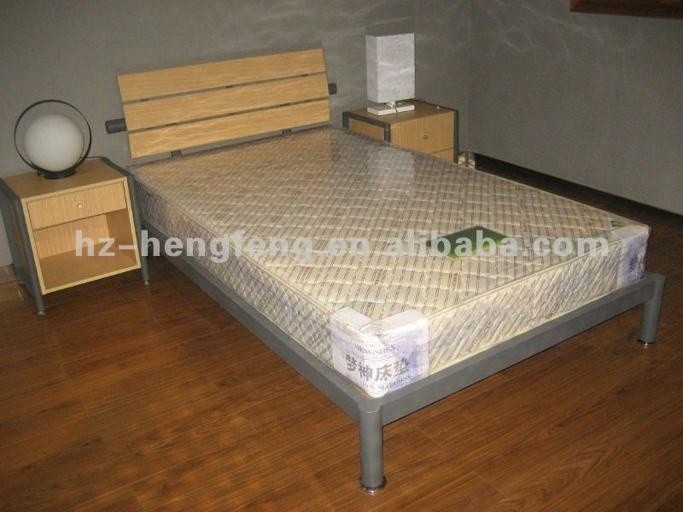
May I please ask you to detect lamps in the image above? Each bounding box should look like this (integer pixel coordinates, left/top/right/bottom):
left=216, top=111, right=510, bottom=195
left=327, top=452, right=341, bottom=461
left=12, top=97, right=91, bottom=178
left=364, top=30, right=415, bottom=115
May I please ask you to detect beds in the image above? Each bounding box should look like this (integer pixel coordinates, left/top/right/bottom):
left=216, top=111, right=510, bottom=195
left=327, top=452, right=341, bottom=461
left=103, top=46, right=667, bottom=493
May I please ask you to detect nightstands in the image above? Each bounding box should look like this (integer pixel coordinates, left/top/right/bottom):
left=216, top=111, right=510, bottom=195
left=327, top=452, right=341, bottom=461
left=344, top=97, right=459, bottom=162
left=0, top=155, right=150, bottom=315
